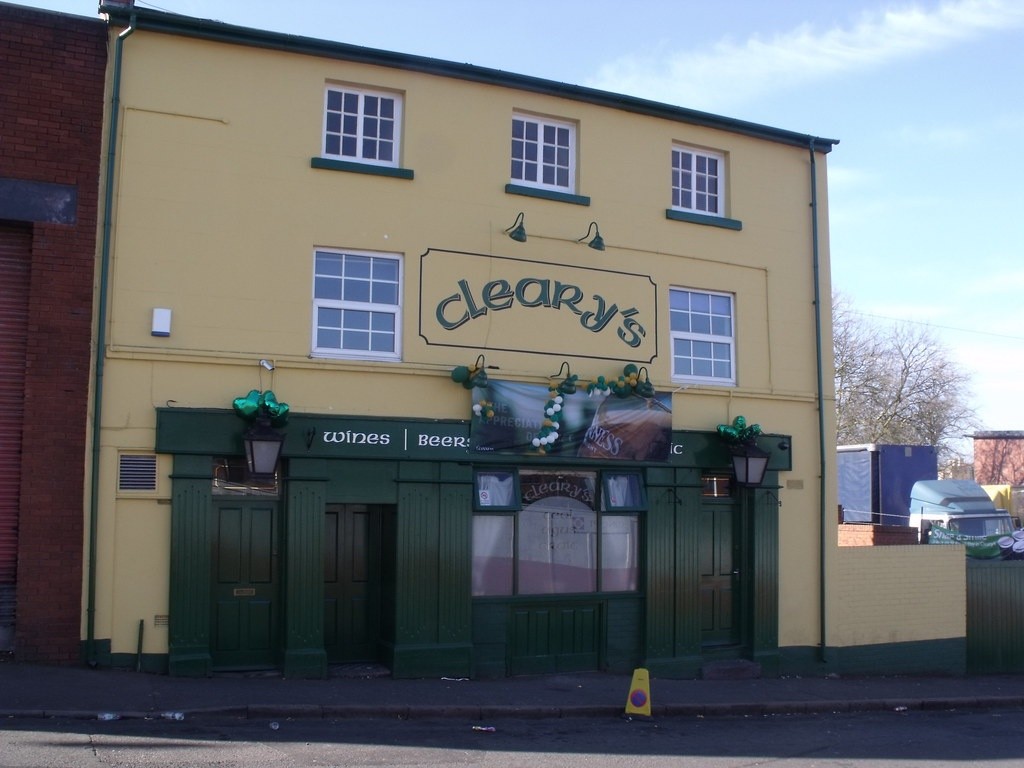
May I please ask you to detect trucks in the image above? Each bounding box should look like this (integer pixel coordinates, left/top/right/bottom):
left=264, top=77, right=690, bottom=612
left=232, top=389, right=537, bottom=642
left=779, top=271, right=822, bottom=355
left=836, top=442, right=1023, bottom=545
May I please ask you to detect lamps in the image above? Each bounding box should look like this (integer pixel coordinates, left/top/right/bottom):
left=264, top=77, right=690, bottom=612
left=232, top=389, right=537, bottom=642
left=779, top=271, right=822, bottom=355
left=732, top=440, right=783, bottom=490
left=505, top=212, right=526, bottom=242
left=636, top=367, right=655, bottom=398
left=578, top=221, right=606, bottom=251
left=550, top=361, right=576, bottom=394
left=462, top=354, right=487, bottom=390
left=241, top=415, right=288, bottom=476
